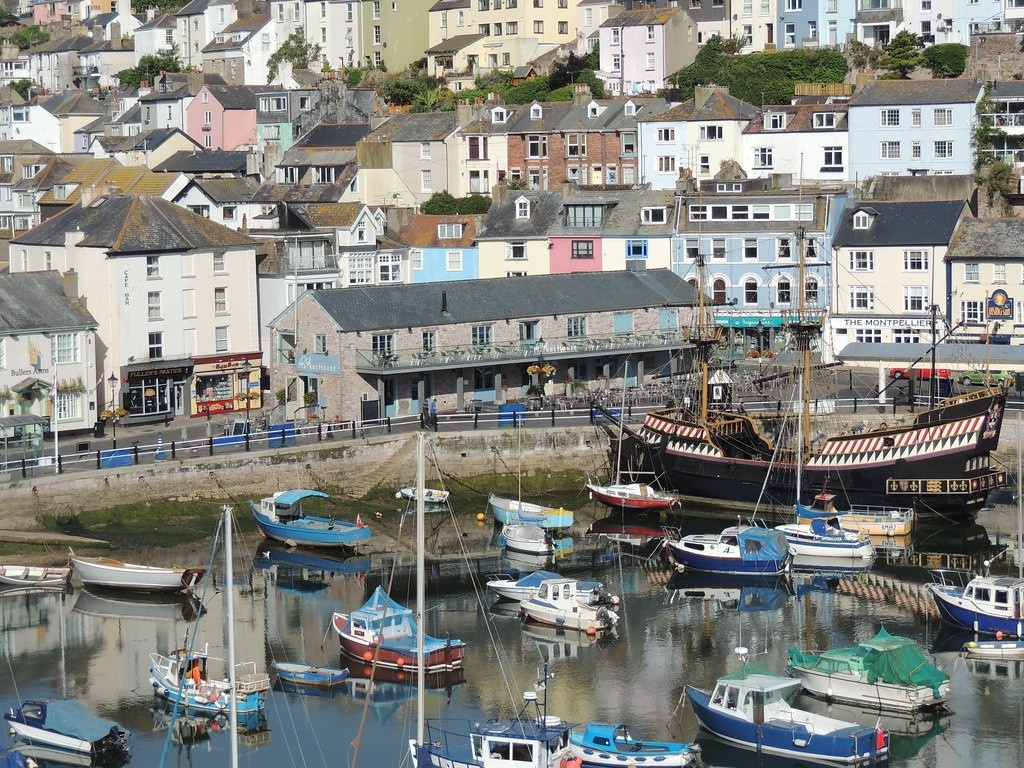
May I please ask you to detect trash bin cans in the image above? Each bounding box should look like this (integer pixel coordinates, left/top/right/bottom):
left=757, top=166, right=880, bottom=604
left=268, top=423, right=296, bottom=448
left=93, top=422, right=104, bottom=437
left=498, top=403, right=524, bottom=427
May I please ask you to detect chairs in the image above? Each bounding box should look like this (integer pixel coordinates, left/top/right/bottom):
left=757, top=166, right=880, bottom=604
left=249, top=417, right=266, bottom=437
left=371, top=334, right=781, bottom=407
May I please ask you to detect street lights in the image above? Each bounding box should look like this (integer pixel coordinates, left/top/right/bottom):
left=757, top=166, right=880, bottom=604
left=242, top=357, right=253, bottom=452
left=755, top=320, right=765, bottom=395
left=536, top=335, right=547, bottom=411
left=108, top=371, right=118, bottom=449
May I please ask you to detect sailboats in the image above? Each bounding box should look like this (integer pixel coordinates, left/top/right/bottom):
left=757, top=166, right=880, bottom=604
left=590, top=151, right=1010, bottom=523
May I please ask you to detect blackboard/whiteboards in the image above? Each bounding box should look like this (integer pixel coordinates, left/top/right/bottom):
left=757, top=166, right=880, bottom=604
left=360, top=401, right=381, bottom=424
left=233, top=423, right=244, bottom=434
left=321, top=424, right=334, bottom=439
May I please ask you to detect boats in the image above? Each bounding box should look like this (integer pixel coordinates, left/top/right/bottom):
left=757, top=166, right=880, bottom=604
left=685, top=645, right=890, bottom=764
left=498, top=512, right=558, bottom=555
left=333, top=585, right=466, bottom=674
left=571, top=721, right=702, bottom=768
left=338, top=652, right=468, bottom=725
left=271, top=657, right=349, bottom=686
left=520, top=578, right=620, bottom=632
left=521, top=612, right=617, bottom=668
left=252, top=537, right=372, bottom=576
left=773, top=522, right=877, bottom=558
left=147, top=695, right=272, bottom=758
left=783, top=625, right=951, bottom=711
left=586, top=514, right=676, bottom=548
left=928, top=624, right=1024, bottom=683
left=408, top=653, right=583, bottom=768
left=67, top=545, right=207, bottom=593
left=660, top=514, right=796, bottom=577
left=272, top=677, right=349, bottom=699
left=1, top=737, right=131, bottom=768
left=485, top=569, right=604, bottom=606
left=5, top=696, right=132, bottom=753
left=333, top=584, right=466, bottom=670
left=923, top=568, right=1024, bottom=641
left=665, top=568, right=794, bottom=615
left=963, top=641, right=1024, bottom=656
left=791, top=687, right=958, bottom=763
left=499, top=545, right=556, bottom=573
left=793, top=477, right=915, bottom=536
left=485, top=529, right=574, bottom=561
left=0, top=565, right=74, bottom=586
left=793, top=555, right=876, bottom=587
left=250, top=488, right=374, bottom=548
left=487, top=597, right=616, bottom=622
left=147, top=626, right=271, bottom=714
left=488, top=414, right=575, bottom=529
left=869, top=536, right=915, bottom=559
left=72, top=586, right=207, bottom=623
left=585, top=360, right=679, bottom=511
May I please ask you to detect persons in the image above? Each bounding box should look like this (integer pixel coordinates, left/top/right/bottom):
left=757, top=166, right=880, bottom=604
left=223, top=417, right=230, bottom=435
left=422, top=398, right=437, bottom=429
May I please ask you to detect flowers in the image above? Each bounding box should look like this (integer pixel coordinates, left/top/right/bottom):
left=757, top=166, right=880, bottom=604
left=99, top=407, right=127, bottom=420
left=527, top=364, right=542, bottom=375
left=235, top=392, right=259, bottom=401
left=747, top=349, right=759, bottom=357
left=542, top=363, right=557, bottom=376
left=764, top=349, right=773, bottom=357
